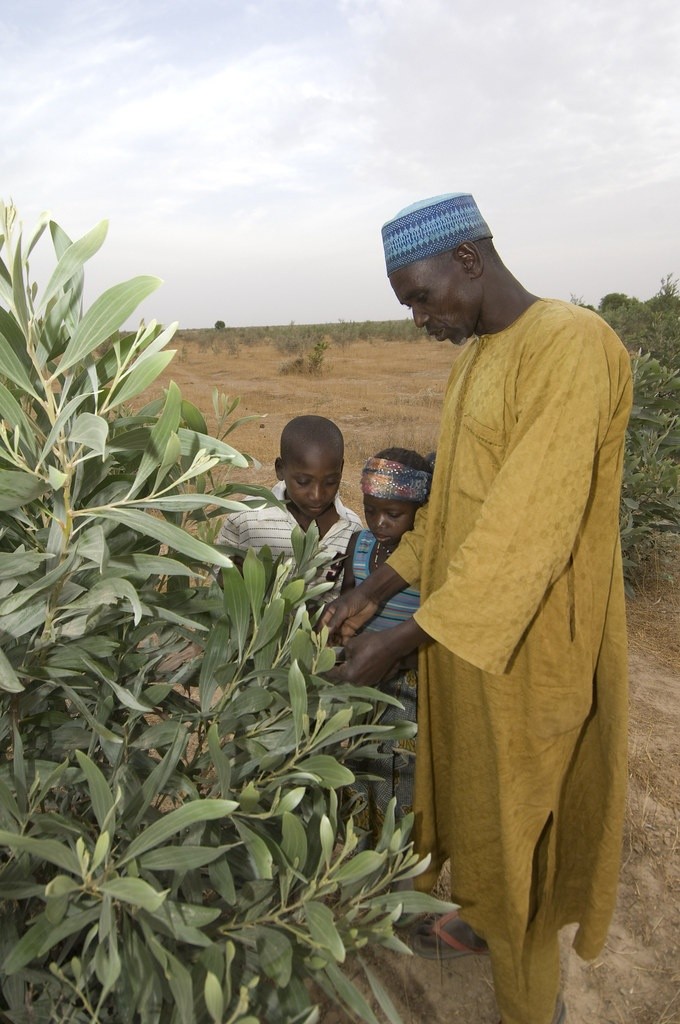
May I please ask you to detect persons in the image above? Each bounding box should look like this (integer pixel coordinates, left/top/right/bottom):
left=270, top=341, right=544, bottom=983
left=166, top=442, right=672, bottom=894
left=208, top=415, right=363, bottom=619
left=313, top=194, right=634, bottom=1024
left=336, top=448, right=435, bottom=928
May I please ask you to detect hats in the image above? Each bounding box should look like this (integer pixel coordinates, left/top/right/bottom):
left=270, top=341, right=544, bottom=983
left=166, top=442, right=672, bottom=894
left=381, top=193, right=494, bottom=276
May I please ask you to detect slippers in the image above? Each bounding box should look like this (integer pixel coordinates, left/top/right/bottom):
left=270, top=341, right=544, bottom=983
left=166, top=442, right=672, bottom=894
left=411, top=912, right=490, bottom=956
